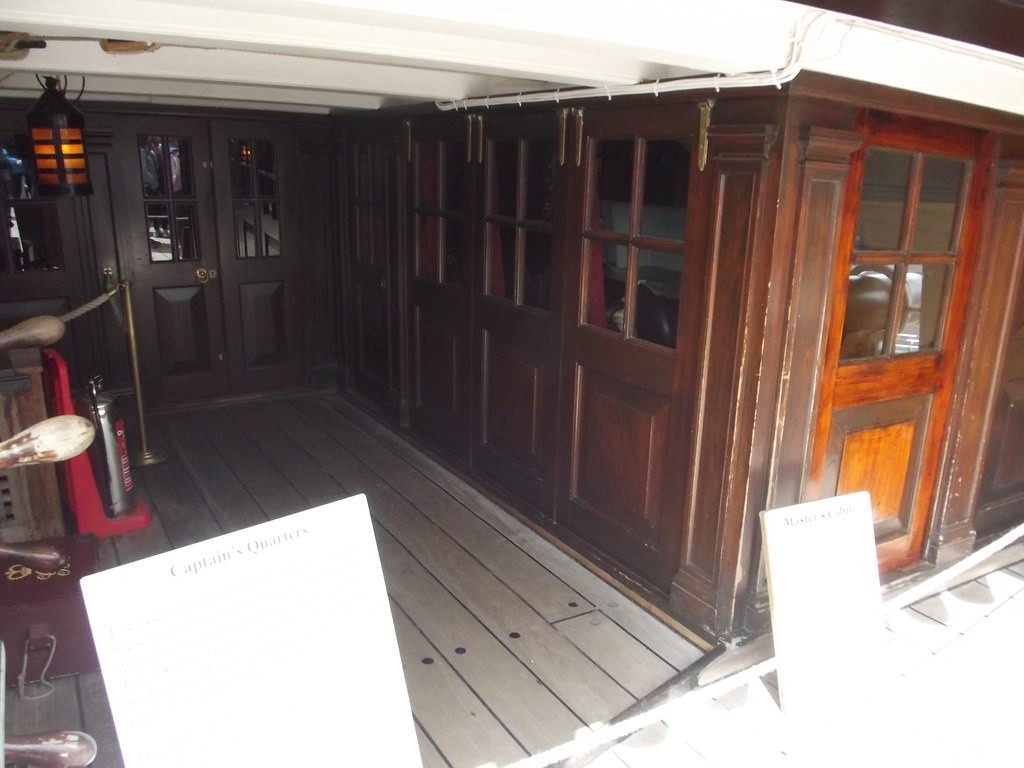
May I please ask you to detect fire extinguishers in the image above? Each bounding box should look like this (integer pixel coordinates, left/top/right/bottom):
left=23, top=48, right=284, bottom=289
left=79, top=374, right=137, bottom=519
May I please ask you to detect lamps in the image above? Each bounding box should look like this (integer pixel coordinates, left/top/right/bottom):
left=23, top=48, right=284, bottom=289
left=26, top=72, right=94, bottom=198
